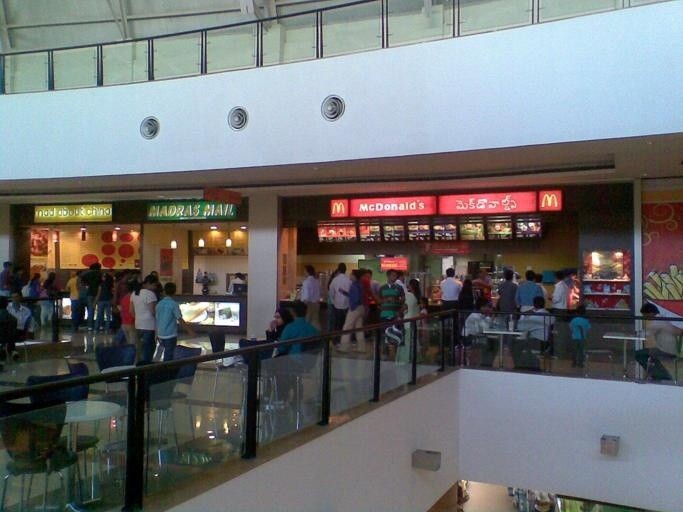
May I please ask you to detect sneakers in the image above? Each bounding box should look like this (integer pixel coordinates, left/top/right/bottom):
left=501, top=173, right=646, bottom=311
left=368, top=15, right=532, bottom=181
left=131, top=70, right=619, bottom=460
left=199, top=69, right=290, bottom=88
left=76, top=324, right=114, bottom=332
left=11, top=350, right=20, bottom=360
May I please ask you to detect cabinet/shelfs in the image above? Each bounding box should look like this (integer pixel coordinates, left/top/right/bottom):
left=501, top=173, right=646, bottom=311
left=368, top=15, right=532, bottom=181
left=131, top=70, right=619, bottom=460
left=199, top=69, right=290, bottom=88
left=582, top=276, right=631, bottom=311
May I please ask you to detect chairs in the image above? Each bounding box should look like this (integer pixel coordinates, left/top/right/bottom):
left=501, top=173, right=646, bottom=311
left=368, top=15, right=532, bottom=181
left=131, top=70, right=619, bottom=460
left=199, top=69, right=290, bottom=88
left=0, top=327, right=333, bottom=511
left=641, top=327, right=683, bottom=384
left=572, top=322, right=618, bottom=380
left=446, top=310, right=477, bottom=367
left=522, top=320, right=558, bottom=374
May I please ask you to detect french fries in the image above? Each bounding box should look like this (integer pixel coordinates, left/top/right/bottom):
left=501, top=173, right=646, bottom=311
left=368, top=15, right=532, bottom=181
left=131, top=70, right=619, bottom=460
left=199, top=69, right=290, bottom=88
left=643, top=265, right=683, bottom=300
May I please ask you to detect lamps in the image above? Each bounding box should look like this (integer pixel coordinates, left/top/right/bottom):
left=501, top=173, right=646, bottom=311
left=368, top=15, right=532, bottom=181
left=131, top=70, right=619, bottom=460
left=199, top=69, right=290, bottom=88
left=170, top=223, right=177, bottom=249
left=197, top=222, right=205, bottom=248
left=48, top=222, right=120, bottom=243
left=224, top=221, right=232, bottom=248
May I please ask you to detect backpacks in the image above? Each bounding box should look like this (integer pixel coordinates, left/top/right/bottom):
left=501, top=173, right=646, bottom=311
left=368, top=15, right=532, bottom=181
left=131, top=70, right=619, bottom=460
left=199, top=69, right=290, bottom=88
left=21, top=279, right=32, bottom=298
left=40, top=288, right=48, bottom=299
